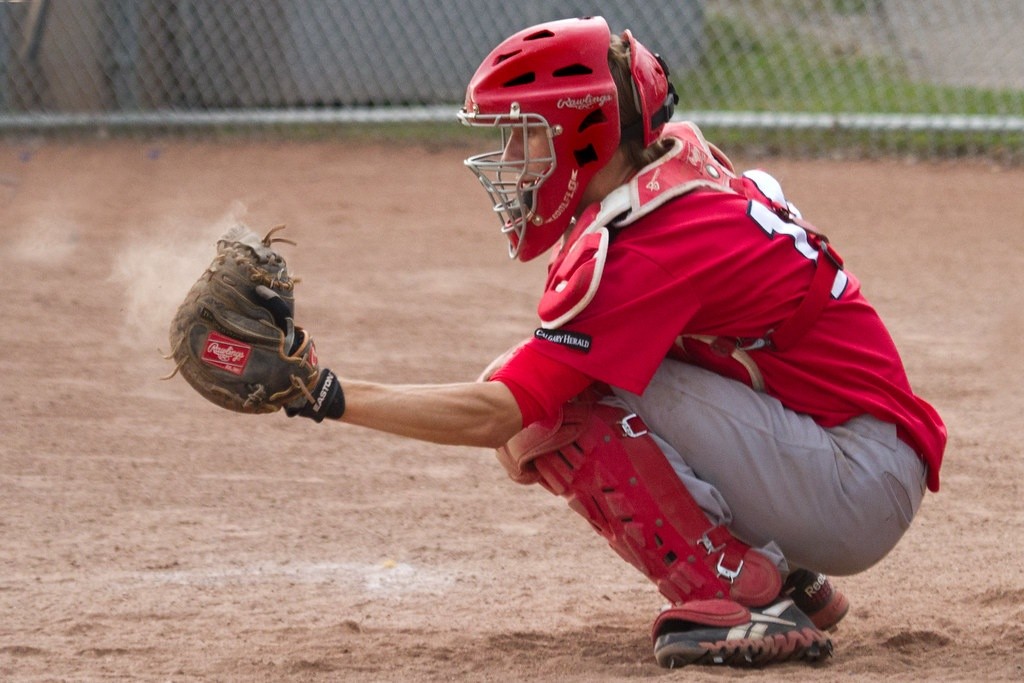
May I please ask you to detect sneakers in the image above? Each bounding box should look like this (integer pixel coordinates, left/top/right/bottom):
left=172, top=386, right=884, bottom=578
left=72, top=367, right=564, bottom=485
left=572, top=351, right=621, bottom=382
left=654, top=597, right=834, bottom=668
left=749, top=569, right=850, bottom=629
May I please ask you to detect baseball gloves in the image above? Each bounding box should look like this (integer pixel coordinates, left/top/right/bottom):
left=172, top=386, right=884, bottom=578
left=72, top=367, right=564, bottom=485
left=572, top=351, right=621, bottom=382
left=161, top=220, right=319, bottom=414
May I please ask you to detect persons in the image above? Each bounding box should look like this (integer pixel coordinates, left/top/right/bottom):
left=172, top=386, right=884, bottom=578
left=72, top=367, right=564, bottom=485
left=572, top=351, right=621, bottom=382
left=157, top=14, right=949, bottom=668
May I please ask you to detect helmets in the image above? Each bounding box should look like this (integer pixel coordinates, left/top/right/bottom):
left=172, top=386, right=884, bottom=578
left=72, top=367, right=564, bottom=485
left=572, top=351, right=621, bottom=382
left=457, top=15, right=680, bottom=262
